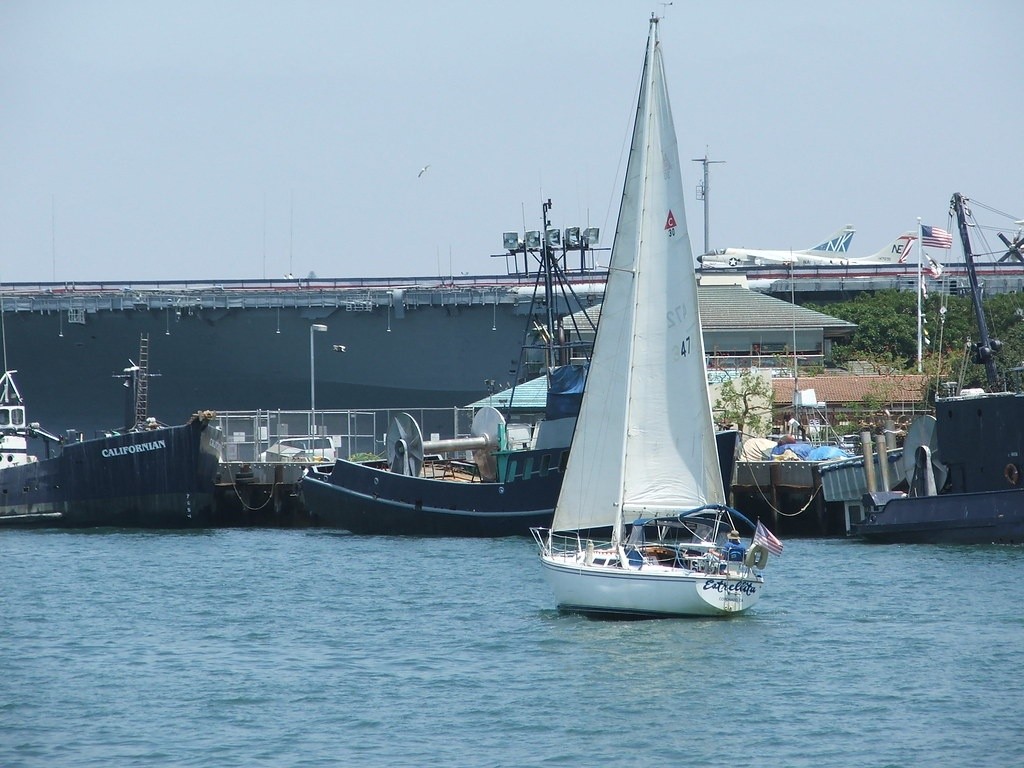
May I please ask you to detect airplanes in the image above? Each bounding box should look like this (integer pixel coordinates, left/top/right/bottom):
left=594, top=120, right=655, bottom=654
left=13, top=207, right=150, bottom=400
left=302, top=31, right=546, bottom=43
left=696, top=224, right=920, bottom=267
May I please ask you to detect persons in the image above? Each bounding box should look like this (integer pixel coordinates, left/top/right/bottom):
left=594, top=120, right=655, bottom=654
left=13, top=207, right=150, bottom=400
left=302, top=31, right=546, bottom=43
left=709, top=530, right=744, bottom=574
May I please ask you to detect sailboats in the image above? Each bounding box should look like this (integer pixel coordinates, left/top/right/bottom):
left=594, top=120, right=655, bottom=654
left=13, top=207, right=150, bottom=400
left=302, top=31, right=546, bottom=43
left=525, top=6, right=786, bottom=618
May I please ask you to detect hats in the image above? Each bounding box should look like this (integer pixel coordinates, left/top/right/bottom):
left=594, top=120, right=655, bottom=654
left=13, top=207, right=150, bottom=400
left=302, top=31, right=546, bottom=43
left=727, top=531, right=740, bottom=540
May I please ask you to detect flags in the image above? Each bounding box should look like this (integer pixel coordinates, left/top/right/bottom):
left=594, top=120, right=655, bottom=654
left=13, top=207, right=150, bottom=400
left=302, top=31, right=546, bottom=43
left=921, top=275, right=928, bottom=298
left=754, top=521, right=783, bottom=557
left=922, top=314, right=930, bottom=344
left=922, top=253, right=942, bottom=278
left=922, top=225, right=952, bottom=248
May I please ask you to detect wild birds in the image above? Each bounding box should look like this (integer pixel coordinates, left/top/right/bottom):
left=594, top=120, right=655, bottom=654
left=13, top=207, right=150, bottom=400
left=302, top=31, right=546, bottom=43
left=418, top=164, right=431, bottom=179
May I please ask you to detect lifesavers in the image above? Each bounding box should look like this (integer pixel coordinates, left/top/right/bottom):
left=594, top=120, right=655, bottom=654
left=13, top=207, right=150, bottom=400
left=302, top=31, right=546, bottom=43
left=744, top=541, right=769, bottom=570
left=1004, top=463, right=1020, bottom=486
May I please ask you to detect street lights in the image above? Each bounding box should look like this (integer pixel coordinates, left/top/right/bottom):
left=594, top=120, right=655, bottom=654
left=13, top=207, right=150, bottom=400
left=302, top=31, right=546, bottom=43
left=310, top=324, right=327, bottom=459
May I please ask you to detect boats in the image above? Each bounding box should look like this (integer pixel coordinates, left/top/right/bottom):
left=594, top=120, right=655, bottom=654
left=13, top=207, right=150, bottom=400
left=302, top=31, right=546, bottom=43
left=1, top=294, right=598, bottom=536
left=714, top=190, right=1023, bottom=547
